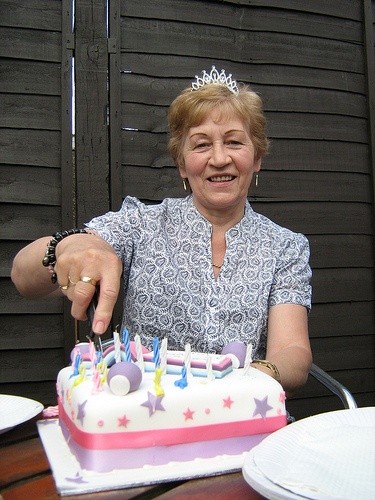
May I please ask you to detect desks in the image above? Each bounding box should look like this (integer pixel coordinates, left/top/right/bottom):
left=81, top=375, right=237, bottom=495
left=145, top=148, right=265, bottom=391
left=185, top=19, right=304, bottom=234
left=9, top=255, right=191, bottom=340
left=0, top=415, right=270, bottom=500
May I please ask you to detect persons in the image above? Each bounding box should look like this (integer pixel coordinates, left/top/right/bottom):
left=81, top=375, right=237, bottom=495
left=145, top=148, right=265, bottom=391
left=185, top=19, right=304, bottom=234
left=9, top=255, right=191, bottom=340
left=11, top=65, right=313, bottom=389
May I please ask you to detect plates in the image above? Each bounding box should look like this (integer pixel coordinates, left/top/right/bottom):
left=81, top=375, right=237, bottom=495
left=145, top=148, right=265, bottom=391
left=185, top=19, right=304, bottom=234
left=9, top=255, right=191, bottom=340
left=0, top=395, right=45, bottom=433
left=242, top=406, right=375, bottom=500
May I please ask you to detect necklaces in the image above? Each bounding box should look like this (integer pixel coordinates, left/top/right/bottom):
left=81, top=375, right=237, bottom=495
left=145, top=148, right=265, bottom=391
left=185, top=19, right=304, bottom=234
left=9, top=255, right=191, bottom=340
left=213, top=263, right=221, bottom=268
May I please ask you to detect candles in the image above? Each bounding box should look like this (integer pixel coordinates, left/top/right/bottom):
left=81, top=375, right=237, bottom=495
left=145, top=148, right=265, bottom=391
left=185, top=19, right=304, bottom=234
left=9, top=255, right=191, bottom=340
left=160, top=336, right=167, bottom=374
left=134, top=330, right=144, bottom=371
left=113, top=324, right=121, bottom=363
left=87, top=336, right=107, bottom=391
left=184, top=343, right=192, bottom=377
left=122, top=326, right=131, bottom=362
left=244, top=343, right=252, bottom=376
left=174, top=362, right=188, bottom=389
left=206, top=355, right=216, bottom=383
left=154, top=367, right=164, bottom=396
left=73, top=351, right=85, bottom=384
left=153, top=335, right=160, bottom=369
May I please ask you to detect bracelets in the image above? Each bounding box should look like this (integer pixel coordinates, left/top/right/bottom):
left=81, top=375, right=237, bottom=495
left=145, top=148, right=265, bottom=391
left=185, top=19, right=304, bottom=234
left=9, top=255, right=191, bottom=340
left=43, top=228, right=97, bottom=282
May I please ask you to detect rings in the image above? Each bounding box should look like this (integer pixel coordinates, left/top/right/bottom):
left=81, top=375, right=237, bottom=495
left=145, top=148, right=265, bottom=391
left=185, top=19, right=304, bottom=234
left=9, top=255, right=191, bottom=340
left=68, top=276, right=79, bottom=286
left=79, top=277, right=97, bottom=287
left=59, top=285, right=71, bottom=290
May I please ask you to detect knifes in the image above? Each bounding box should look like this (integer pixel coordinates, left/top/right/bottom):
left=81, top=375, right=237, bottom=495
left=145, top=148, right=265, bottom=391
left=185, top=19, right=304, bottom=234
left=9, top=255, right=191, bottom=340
left=87, top=297, right=103, bottom=354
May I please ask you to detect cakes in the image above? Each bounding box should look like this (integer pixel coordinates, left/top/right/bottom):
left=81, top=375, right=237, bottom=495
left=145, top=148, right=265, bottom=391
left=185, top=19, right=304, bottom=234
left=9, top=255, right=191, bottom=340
left=56, top=341, right=288, bottom=487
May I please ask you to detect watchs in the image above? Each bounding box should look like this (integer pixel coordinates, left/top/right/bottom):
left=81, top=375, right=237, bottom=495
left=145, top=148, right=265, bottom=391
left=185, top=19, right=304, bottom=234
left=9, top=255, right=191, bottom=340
left=251, top=360, right=281, bottom=383
